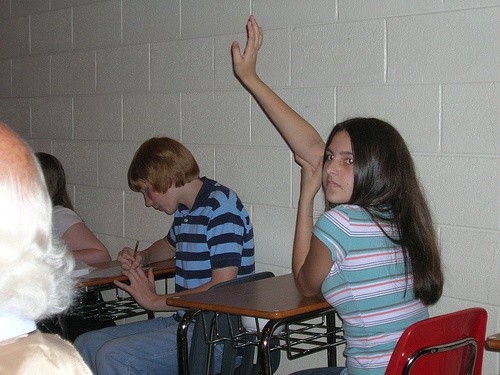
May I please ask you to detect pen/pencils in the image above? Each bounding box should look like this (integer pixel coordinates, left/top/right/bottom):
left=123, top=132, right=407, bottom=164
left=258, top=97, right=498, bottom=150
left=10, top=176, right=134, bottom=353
left=131, top=240, right=140, bottom=267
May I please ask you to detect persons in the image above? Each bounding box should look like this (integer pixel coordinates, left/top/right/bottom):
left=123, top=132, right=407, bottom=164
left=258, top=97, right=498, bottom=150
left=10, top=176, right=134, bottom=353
left=231, top=14, right=444, bottom=375
left=72, top=137, right=255, bottom=375
left=0, top=120, right=96, bottom=375
left=34, top=152, right=112, bottom=270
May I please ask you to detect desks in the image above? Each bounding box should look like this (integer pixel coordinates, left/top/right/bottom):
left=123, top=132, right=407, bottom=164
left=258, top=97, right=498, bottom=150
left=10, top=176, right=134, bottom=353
left=166, top=272, right=348, bottom=375
left=37, top=258, right=179, bottom=340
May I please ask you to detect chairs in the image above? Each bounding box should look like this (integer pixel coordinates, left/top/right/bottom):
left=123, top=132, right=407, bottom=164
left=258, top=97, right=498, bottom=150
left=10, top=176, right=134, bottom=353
left=188, top=272, right=281, bottom=375
left=384, top=307, right=487, bottom=375
